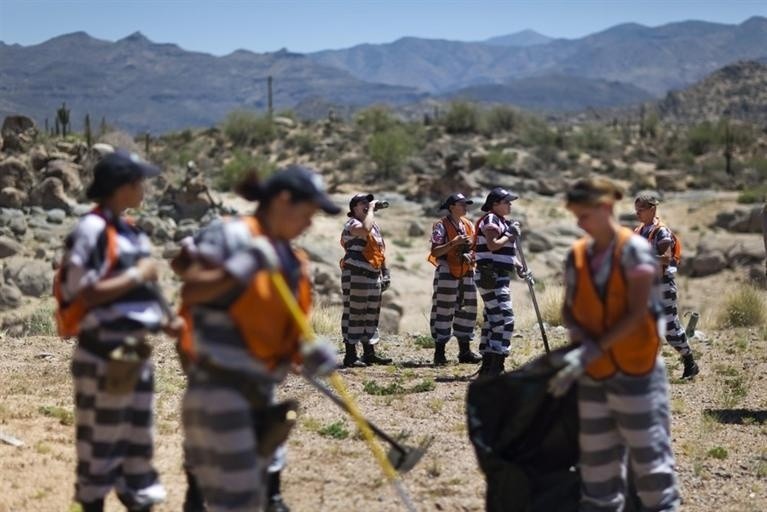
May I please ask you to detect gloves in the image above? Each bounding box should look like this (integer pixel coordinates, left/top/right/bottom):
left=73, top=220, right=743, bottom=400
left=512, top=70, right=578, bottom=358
left=544, top=340, right=602, bottom=400
left=518, top=262, right=534, bottom=285
left=226, top=239, right=281, bottom=280
left=506, top=220, right=521, bottom=241
left=298, top=333, right=338, bottom=377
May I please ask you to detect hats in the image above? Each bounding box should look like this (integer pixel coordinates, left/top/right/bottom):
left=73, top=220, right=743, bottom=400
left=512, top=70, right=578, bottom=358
left=267, top=165, right=341, bottom=216
left=487, top=186, right=518, bottom=204
left=349, top=194, right=373, bottom=210
left=634, top=193, right=658, bottom=209
left=89, top=153, right=161, bottom=197
left=447, top=192, right=472, bottom=206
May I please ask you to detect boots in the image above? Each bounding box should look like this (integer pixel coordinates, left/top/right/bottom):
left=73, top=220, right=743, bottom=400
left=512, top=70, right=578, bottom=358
left=681, top=355, right=700, bottom=381
left=480, top=353, right=507, bottom=378
left=459, top=343, right=484, bottom=363
left=434, top=345, right=447, bottom=363
left=363, top=344, right=392, bottom=365
left=344, top=347, right=366, bottom=367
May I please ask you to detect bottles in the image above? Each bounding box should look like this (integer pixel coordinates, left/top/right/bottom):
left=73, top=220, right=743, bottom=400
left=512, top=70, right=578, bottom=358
left=363, top=199, right=390, bottom=215
left=480, top=263, right=497, bottom=288
left=258, top=398, right=300, bottom=454
left=103, top=337, right=142, bottom=393
left=456, top=228, right=472, bottom=263
left=684, top=311, right=699, bottom=337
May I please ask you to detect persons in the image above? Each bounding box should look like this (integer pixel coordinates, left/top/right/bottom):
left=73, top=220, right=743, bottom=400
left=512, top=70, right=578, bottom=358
left=631, top=193, right=700, bottom=384
left=169, top=163, right=342, bottom=512
left=338, top=190, right=395, bottom=368
left=427, top=193, right=485, bottom=367
left=49, top=142, right=190, bottom=512
left=471, top=188, right=536, bottom=381
left=543, top=177, right=690, bottom=510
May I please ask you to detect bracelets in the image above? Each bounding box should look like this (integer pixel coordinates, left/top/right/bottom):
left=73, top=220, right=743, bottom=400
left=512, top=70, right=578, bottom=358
left=126, top=267, right=145, bottom=287
left=596, top=333, right=613, bottom=355
